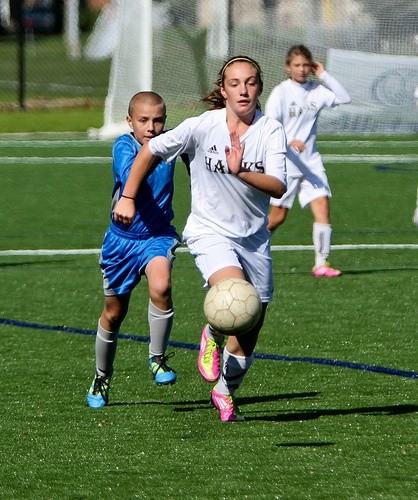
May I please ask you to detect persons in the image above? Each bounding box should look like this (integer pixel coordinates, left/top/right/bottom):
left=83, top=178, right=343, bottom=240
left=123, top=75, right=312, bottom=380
left=265, top=44, right=353, bottom=277
left=87, top=92, right=191, bottom=407
left=115, top=56, right=288, bottom=424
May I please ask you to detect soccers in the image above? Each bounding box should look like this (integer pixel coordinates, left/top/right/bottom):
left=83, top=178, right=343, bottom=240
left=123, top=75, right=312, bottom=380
left=204, top=278, right=263, bottom=335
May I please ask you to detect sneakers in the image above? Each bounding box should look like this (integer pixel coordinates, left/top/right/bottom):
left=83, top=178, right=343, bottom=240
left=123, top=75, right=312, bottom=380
left=87, top=366, right=113, bottom=408
left=312, top=262, right=342, bottom=277
left=197, top=322, right=225, bottom=382
left=211, top=384, right=243, bottom=422
left=148, top=352, right=176, bottom=385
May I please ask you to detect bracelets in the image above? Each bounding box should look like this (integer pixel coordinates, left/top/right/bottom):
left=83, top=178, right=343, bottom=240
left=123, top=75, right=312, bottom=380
left=121, top=195, right=134, bottom=201
